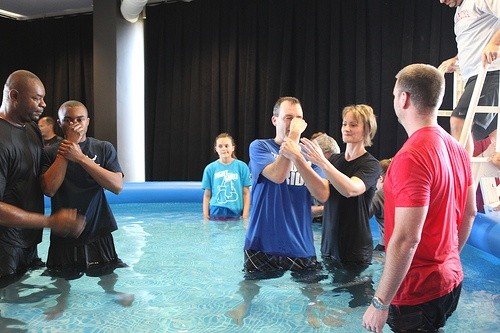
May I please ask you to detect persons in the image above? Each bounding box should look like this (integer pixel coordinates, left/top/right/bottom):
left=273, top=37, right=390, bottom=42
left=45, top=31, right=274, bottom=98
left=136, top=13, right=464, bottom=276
left=37, top=116, right=63, bottom=147
left=306, top=132, right=341, bottom=222
left=0, top=70, right=86, bottom=278
left=43, top=99, right=126, bottom=277
left=300, top=104, right=381, bottom=263
left=435, top=0, right=500, bottom=158
left=369, top=160, right=391, bottom=251
left=362, top=62, right=478, bottom=333
left=201, top=133, right=252, bottom=222
left=241, top=96, right=321, bottom=279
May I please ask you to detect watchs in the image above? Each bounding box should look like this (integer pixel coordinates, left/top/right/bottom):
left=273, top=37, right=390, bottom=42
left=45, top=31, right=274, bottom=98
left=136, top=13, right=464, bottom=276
left=372, top=297, right=390, bottom=310
